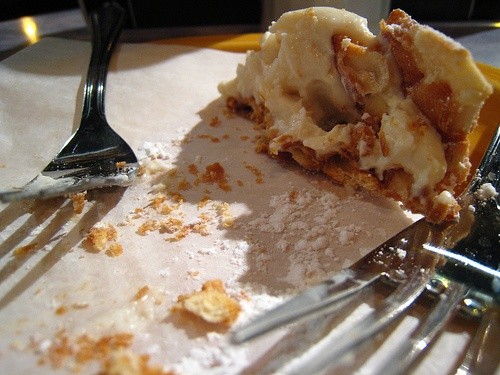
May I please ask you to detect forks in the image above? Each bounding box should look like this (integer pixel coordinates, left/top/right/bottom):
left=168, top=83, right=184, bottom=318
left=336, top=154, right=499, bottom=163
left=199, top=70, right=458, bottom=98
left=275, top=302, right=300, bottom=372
left=1, top=1, right=143, bottom=199
left=230, top=66, right=500, bottom=375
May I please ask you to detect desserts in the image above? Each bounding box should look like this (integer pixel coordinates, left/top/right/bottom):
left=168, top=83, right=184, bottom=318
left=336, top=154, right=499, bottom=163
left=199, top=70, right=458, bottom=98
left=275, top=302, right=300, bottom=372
left=216, top=6, right=493, bottom=224
